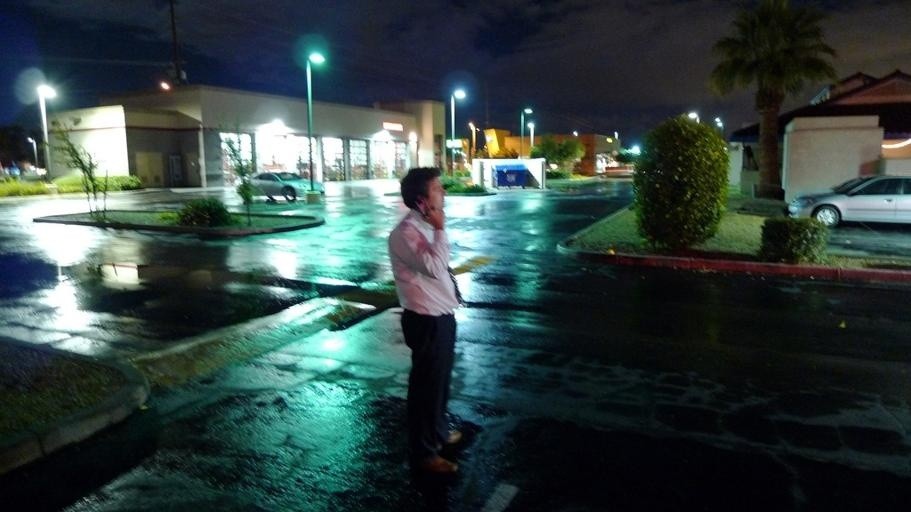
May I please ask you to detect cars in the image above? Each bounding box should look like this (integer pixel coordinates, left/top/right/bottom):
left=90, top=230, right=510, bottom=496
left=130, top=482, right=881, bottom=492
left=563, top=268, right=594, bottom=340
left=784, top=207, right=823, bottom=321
left=789, top=173, right=911, bottom=229
left=236, top=171, right=325, bottom=202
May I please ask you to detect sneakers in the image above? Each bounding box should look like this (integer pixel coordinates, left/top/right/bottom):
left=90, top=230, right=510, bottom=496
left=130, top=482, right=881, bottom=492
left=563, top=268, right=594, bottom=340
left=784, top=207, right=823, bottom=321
left=418, top=454, right=458, bottom=472
left=446, top=426, right=461, bottom=445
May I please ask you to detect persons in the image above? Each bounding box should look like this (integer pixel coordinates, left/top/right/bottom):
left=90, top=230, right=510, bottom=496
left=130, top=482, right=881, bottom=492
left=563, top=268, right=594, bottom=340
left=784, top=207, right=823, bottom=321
left=387, top=163, right=466, bottom=476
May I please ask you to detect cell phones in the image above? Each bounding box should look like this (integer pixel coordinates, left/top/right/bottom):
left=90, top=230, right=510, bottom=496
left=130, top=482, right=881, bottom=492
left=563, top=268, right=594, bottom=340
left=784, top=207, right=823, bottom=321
left=413, top=192, right=431, bottom=218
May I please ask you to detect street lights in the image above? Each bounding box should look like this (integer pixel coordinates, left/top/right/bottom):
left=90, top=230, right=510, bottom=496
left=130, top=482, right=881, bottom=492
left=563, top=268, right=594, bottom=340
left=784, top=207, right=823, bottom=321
left=452, top=89, right=467, bottom=180
left=519, top=108, right=533, bottom=158
left=528, top=121, right=535, bottom=148
left=467, top=121, right=478, bottom=158
left=26, top=135, right=40, bottom=171
left=38, top=85, right=57, bottom=180
left=304, top=52, right=326, bottom=205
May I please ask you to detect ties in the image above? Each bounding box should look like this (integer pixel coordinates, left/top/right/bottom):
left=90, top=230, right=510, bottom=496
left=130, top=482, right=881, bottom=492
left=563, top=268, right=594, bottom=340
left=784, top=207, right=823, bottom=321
left=446, top=265, right=465, bottom=306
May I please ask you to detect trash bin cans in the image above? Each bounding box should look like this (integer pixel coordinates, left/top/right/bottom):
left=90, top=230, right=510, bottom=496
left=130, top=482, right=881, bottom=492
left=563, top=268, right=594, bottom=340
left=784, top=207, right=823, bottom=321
left=492, top=165, right=527, bottom=189
